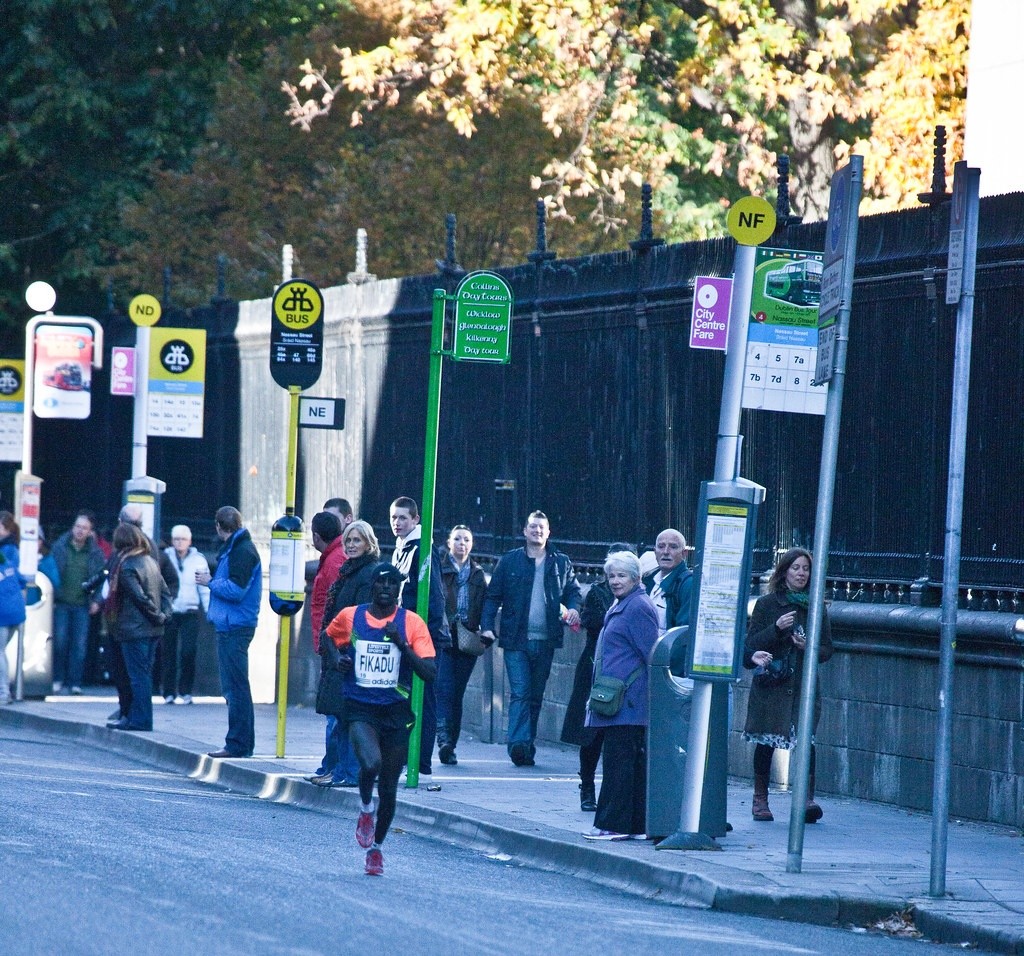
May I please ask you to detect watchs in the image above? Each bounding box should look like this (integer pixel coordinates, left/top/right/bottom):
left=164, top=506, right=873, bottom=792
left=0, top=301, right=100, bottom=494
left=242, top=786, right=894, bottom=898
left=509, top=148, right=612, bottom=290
left=400, top=641, right=409, bottom=651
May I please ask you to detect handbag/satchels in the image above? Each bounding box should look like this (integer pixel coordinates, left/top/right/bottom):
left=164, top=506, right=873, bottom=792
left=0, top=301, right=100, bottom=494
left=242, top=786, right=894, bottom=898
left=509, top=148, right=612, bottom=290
left=456, top=621, right=484, bottom=656
left=588, top=676, right=625, bottom=716
left=752, top=659, right=790, bottom=690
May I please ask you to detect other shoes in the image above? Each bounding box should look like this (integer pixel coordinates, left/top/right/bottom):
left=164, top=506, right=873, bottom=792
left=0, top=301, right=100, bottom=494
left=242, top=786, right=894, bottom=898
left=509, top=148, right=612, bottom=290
left=398, top=770, right=432, bottom=783
left=184, top=695, right=194, bottom=705
left=69, top=685, right=82, bottom=695
left=305, top=773, right=357, bottom=787
left=511, top=743, right=536, bottom=767
left=164, top=695, right=175, bottom=705
left=53, top=682, right=62, bottom=693
left=438, top=744, right=458, bottom=765
left=106, top=716, right=130, bottom=728
left=117, top=723, right=137, bottom=730
left=0, top=693, right=14, bottom=706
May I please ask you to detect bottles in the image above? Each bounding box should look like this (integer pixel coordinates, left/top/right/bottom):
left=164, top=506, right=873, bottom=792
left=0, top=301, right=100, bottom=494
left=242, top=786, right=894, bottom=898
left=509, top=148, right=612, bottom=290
left=559, top=610, right=584, bottom=634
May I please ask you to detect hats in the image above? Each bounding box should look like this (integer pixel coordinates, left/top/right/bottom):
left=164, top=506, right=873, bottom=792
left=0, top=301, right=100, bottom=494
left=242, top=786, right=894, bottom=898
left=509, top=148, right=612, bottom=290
left=371, top=561, right=400, bottom=591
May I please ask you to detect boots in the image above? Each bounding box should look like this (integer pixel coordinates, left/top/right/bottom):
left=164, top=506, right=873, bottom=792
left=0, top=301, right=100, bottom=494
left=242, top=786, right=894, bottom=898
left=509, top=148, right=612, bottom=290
left=751, top=773, right=774, bottom=821
left=578, top=769, right=597, bottom=811
left=805, top=775, right=823, bottom=824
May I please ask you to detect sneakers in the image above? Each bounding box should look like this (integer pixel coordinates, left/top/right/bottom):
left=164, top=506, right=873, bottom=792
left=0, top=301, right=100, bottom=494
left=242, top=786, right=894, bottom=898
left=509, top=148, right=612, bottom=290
left=355, top=809, right=375, bottom=848
left=581, top=826, right=652, bottom=841
left=364, top=849, right=384, bottom=876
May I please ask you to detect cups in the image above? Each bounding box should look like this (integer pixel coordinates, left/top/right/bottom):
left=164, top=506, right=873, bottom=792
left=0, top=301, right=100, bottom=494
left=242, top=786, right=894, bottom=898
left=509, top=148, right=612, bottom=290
left=196, top=568, right=208, bottom=584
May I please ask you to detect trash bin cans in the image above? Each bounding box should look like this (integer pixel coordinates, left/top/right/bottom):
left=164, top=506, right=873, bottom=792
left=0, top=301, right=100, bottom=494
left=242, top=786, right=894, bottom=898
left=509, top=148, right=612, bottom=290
left=645, top=624, right=728, bottom=839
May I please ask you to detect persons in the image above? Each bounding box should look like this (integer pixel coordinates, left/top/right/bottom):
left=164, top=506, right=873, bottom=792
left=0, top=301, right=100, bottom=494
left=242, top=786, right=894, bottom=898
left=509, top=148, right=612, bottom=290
left=87, top=501, right=180, bottom=733
left=52, top=509, right=112, bottom=695
left=741, top=548, right=833, bottom=824
left=162, top=525, right=211, bottom=706
left=432, top=524, right=491, bottom=766
left=479, top=510, right=584, bottom=767
left=0, top=510, right=39, bottom=706
left=642, top=527, right=696, bottom=636
left=321, top=563, right=436, bottom=876
left=389, top=497, right=445, bottom=774
left=195, top=506, right=263, bottom=758
left=33, top=525, right=61, bottom=589
left=313, top=520, right=387, bottom=788
left=584, top=551, right=659, bottom=842
left=305, top=495, right=355, bottom=779
left=559, top=541, right=648, bottom=810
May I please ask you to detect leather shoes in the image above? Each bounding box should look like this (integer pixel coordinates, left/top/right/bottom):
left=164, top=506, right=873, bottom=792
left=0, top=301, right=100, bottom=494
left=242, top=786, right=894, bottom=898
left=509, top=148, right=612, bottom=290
left=207, top=749, right=249, bottom=758
